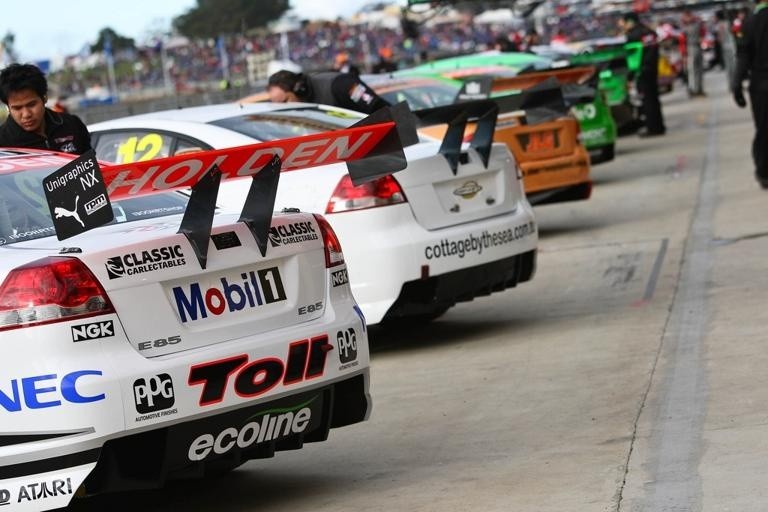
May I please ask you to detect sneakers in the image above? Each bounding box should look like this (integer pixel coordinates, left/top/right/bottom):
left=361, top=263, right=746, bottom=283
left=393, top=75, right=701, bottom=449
left=638, top=125, right=665, bottom=136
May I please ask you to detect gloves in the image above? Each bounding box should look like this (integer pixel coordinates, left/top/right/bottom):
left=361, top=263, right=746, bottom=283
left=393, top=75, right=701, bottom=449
left=734, top=88, right=746, bottom=108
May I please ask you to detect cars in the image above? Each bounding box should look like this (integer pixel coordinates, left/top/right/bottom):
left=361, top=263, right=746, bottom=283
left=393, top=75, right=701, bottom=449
left=397, top=23, right=721, bottom=164
left=233, top=74, right=597, bottom=206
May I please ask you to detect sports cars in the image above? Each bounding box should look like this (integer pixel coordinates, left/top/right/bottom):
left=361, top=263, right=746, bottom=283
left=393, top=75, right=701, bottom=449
left=89, top=96, right=539, bottom=334
left=1, top=116, right=394, bottom=507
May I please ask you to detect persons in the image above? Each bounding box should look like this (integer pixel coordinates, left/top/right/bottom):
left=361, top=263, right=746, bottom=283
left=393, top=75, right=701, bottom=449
left=617, top=10, right=666, bottom=138
left=477, top=9, right=622, bottom=52
left=729, top=5, right=766, bottom=191
left=657, top=10, right=729, bottom=98
left=55, top=16, right=483, bottom=90
left=0, top=62, right=96, bottom=155
left=267, top=69, right=392, bottom=117
